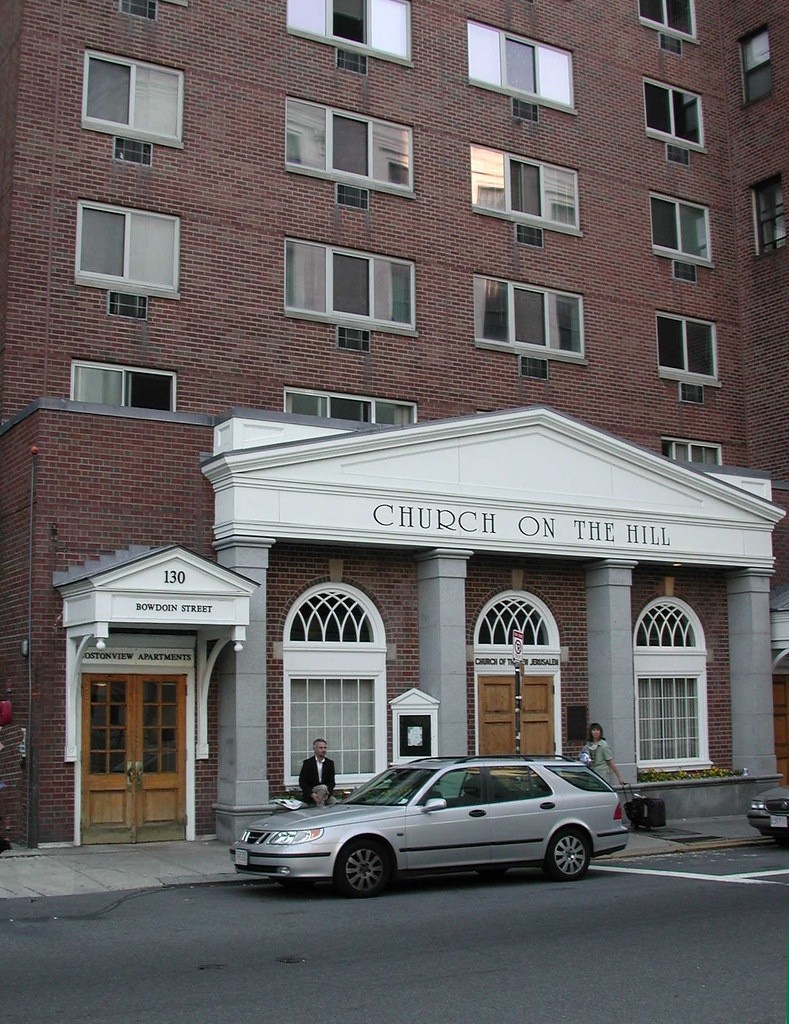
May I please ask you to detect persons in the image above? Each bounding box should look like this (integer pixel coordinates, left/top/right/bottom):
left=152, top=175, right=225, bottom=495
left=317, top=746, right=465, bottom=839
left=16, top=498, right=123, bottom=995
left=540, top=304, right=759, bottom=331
left=299, top=739, right=336, bottom=805
left=579, top=722, right=625, bottom=788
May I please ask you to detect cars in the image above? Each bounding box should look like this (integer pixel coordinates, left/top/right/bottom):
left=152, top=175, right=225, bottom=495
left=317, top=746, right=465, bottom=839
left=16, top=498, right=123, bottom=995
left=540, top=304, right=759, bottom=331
left=748, top=782, right=789, bottom=854
left=228, top=753, right=630, bottom=898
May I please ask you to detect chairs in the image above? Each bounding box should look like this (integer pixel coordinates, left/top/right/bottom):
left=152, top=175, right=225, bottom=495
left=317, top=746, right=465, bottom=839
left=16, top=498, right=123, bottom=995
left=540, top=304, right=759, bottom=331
left=458, top=780, right=480, bottom=806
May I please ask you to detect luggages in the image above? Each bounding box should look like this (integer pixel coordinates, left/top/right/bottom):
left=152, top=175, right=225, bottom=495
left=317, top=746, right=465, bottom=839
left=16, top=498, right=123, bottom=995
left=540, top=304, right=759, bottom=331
left=621, top=782, right=666, bottom=831
